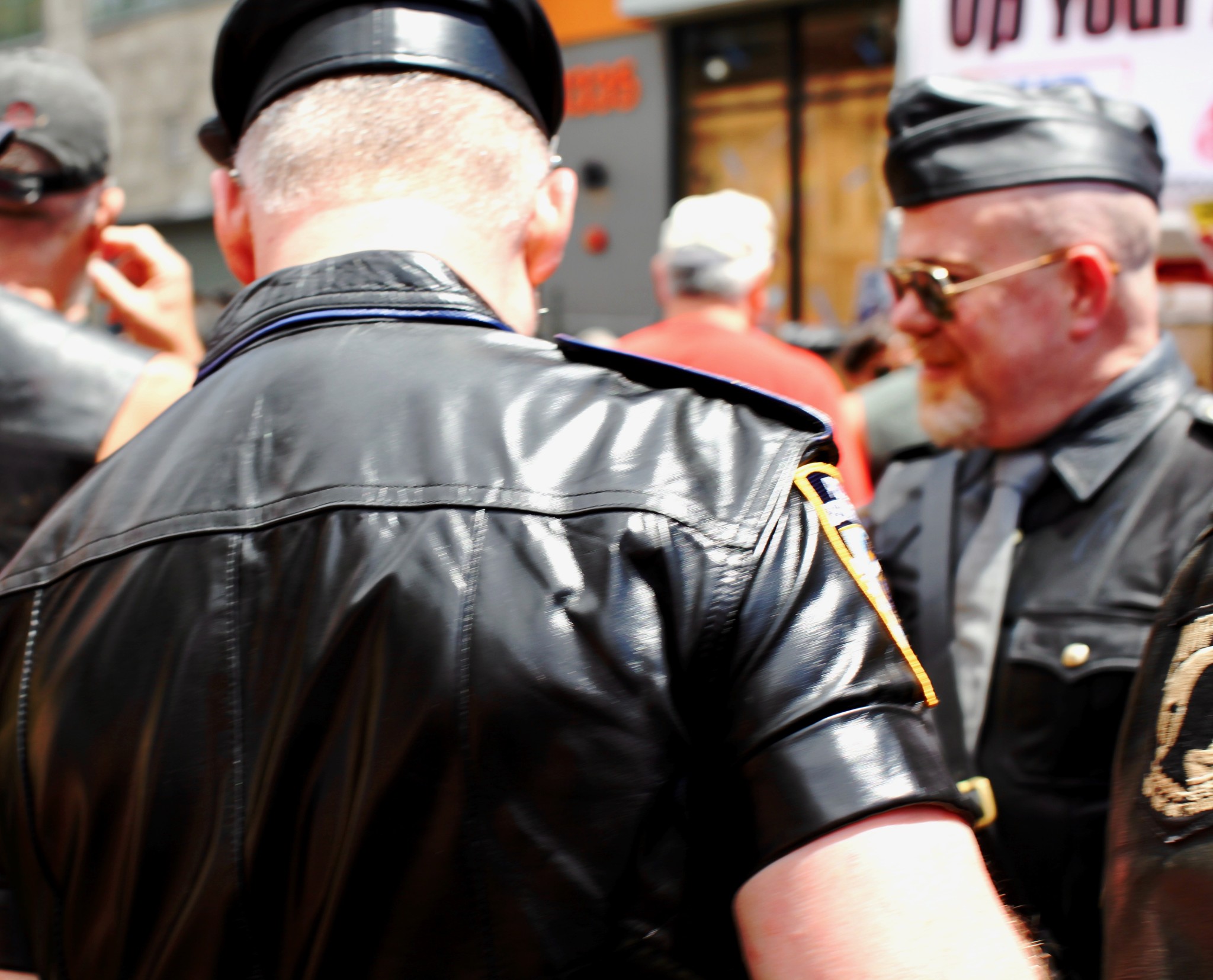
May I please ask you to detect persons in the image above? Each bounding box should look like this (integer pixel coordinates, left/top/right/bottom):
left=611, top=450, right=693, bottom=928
left=0, top=1, right=1050, bottom=980
left=866, top=71, right=1213, bottom=980
left=1, top=39, right=923, bottom=579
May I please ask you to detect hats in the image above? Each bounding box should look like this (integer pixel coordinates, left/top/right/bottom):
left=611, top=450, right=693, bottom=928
left=663, top=190, right=770, bottom=290
left=0, top=47, right=117, bottom=199
left=883, top=76, right=1165, bottom=207
left=197, top=0, right=565, bottom=164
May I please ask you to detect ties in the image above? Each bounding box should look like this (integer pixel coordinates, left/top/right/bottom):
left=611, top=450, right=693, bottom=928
left=950, top=453, right=1048, bottom=765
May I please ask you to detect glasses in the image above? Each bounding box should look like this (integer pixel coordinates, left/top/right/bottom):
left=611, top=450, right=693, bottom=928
left=882, top=246, right=1121, bottom=319
